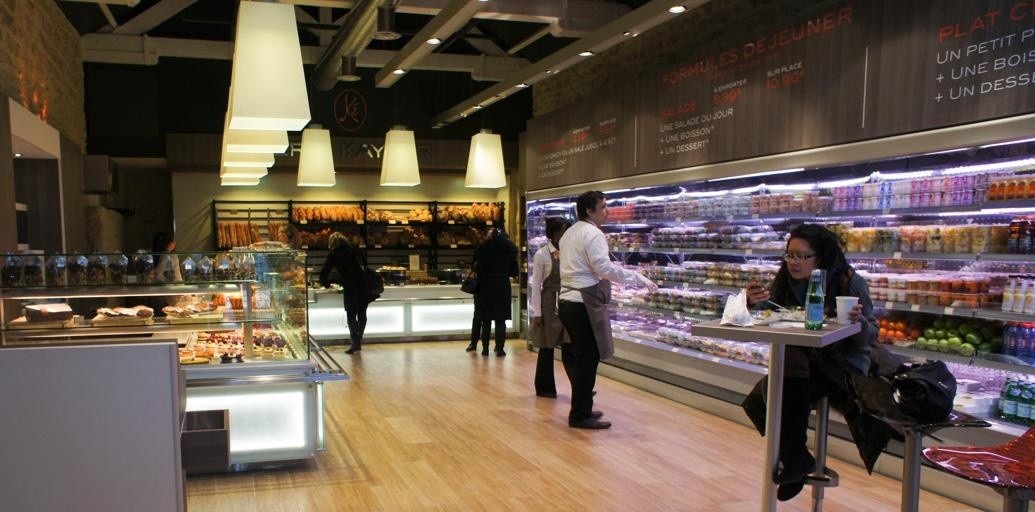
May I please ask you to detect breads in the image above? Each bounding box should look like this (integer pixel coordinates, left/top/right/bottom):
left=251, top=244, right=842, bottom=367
left=218, top=201, right=502, bottom=249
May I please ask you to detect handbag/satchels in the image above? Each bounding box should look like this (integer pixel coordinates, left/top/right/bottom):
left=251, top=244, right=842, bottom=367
left=364, top=269, right=384, bottom=293
left=461, top=276, right=477, bottom=294
left=889, top=359, right=957, bottom=423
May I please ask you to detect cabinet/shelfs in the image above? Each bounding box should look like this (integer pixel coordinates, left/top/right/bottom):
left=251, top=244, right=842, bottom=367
left=515, top=106, right=1034, bottom=512
left=202, top=190, right=510, bottom=285
left=2, top=247, right=356, bottom=476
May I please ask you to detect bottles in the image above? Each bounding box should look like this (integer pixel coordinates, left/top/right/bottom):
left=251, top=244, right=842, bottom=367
left=804, top=269, right=824, bottom=331
left=1002, top=321, right=1035, bottom=361
left=1008, top=213, right=1034, bottom=253
left=1000, top=272, right=1035, bottom=316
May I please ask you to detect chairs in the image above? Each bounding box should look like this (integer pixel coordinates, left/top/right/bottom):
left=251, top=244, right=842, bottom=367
left=916, top=409, right=1034, bottom=510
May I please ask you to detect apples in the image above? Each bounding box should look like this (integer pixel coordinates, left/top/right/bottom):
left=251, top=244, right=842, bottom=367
left=916, top=317, right=1004, bottom=357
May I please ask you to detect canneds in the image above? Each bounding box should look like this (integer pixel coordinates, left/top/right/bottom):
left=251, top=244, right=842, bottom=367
left=631, top=168, right=1035, bottom=221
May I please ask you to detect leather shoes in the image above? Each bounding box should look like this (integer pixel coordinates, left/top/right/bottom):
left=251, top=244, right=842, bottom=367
left=571, top=411, right=611, bottom=429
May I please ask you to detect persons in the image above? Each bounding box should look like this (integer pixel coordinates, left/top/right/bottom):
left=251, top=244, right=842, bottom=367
left=742, top=224, right=881, bottom=502
left=528, top=218, right=572, bottom=399
left=471, top=227, right=519, bottom=356
left=465, top=227, right=498, bottom=351
left=319, top=232, right=369, bottom=353
left=558, top=191, right=660, bottom=429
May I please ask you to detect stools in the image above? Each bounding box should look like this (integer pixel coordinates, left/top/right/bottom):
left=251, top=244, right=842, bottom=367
left=854, top=393, right=997, bottom=509
left=787, top=375, right=845, bottom=511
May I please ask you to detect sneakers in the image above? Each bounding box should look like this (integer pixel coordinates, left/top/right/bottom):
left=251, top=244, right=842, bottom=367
left=776, top=448, right=816, bottom=501
left=467, top=342, right=505, bottom=356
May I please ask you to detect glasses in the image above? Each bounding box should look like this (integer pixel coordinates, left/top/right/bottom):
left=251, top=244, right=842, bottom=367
left=784, top=253, right=816, bottom=264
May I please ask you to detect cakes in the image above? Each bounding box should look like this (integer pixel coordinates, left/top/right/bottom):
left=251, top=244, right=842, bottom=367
left=24, top=294, right=287, bottom=365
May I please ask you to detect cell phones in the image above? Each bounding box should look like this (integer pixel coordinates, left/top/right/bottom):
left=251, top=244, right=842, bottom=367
left=751, top=285, right=764, bottom=294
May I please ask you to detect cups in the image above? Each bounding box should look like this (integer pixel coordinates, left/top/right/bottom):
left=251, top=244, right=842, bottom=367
left=836, top=296, right=859, bottom=325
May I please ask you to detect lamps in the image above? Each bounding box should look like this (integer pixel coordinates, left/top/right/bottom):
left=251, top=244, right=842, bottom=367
left=207, top=0, right=514, bottom=194
